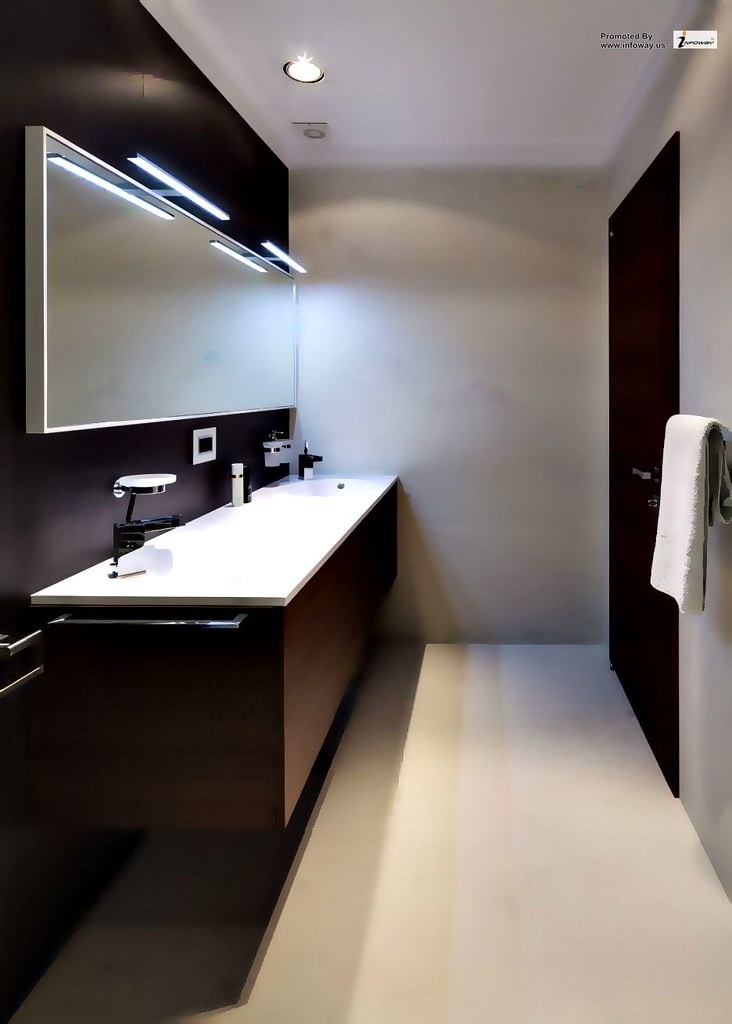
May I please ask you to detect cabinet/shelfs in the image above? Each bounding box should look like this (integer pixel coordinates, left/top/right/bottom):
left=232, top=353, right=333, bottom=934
left=28, top=474, right=400, bottom=837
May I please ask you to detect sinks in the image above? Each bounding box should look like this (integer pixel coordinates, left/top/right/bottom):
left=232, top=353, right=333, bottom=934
left=146, top=519, right=305, bottom=591
left=273, top=478, right=373, bottom=517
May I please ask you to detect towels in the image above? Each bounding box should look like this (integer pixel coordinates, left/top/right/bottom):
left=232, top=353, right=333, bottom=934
left=649, top=412, right=732, bottom=617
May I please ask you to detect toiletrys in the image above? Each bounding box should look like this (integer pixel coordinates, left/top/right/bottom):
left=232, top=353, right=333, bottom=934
left=230, top=462, right=253, bottom=507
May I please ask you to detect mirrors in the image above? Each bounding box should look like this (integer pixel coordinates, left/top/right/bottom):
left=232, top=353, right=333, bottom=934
left=26, top=124, right=299, bottom=434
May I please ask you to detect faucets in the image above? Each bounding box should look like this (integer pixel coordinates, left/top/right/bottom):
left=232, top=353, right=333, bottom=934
left=112, top=513, right=185, bottom=565
left=298, top=452, right=323, bottom=481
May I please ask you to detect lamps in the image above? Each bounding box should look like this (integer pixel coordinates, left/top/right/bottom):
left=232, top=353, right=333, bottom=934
left=46, top=151, right=176, bottom=222
left=259, top=239, right=307, bottom=274
left=209, top=239, right=267, bottom=274
left=125, top=151, right=230, bottom=221
